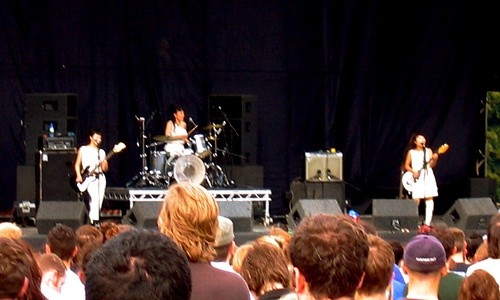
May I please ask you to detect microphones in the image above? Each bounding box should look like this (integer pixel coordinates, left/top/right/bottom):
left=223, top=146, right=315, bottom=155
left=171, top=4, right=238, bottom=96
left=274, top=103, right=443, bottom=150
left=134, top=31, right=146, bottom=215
left=317, top=170, right=321, bottom=177
left=421, top=142, right=425, bottom=148
left=327, top=169, right=331, bottom=176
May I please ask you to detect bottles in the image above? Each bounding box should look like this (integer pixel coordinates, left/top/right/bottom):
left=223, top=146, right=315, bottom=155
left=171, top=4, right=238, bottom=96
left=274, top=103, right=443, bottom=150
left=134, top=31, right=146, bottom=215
left=49, top=123, right=54, bottom=137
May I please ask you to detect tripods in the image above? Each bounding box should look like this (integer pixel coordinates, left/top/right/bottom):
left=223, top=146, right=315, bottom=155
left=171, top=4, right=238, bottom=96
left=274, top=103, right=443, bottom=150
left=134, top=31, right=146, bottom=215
left=125, top=126, right=167, bottom=188
left=205, top=128, right=235, bottom=188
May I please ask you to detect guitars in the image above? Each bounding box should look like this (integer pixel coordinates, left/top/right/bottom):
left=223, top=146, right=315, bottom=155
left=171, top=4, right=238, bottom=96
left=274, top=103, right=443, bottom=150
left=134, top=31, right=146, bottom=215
left=76, top=142, right=126, bottom=192
left=402, top=143, right=450, bottom=192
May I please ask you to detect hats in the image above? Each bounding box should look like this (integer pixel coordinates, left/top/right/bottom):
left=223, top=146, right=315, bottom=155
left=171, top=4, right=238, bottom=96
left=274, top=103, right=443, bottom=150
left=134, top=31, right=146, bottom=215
left=214, top=215, right=235, bottom=247
left=403, top=235, right=446, bottom=270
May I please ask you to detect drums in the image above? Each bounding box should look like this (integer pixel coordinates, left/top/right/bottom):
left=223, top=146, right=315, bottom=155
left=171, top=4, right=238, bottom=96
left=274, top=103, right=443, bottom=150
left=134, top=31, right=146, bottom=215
left=149, top=151, right=170, bottom=179
left=165, top=154, right=206, bottom=186
left=187, top=134, right=211, bottom=159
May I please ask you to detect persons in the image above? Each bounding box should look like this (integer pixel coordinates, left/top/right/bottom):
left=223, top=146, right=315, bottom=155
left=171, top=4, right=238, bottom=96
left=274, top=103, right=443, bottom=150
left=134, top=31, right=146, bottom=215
left=0, top=209, right=500, bottom=300
left=75, top=129, right=108, bottom=229
left=86, top=230, right=192, bottom=300
left=164, top=105, right=194, bottom=157
left=404, top=133, right=438, bottom=227
left=354, top=234, right=395, bottom=300
left=400, top=235, right=448, bottom=300
left=288, top=214, right=369, bottom=300
left=239, top=239, right=299, bottom=300
left=158, top=181, right=250, bottom=300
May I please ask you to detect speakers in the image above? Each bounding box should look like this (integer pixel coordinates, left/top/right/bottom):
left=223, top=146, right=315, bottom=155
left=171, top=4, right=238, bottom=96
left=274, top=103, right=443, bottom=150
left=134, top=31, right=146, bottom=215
left=121, top=202, right=164, bottom=231
left=206, top=95, right=263, bottom=214
left=288, top=199, right=342, bottom=232
left=35, top=200, right=90, bottom=236
left=373, top=199, right=418, bottom=231
left=444, top=198, right=497, bottom=232
left=16, top=93, right=82, bottom=202
left=304, top=180, right=345, bottom=208
left=216, top=201, right=252, bottom=233
left=471, top=178, right=497, bottom=205
left=306, top=152, right=343, bottom=180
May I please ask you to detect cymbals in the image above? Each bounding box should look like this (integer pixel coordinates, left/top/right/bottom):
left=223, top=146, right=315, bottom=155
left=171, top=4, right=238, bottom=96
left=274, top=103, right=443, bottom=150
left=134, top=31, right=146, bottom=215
left=153, top=135, right=170, bottom=140
left=205, top=124, right=223, bottom=130
